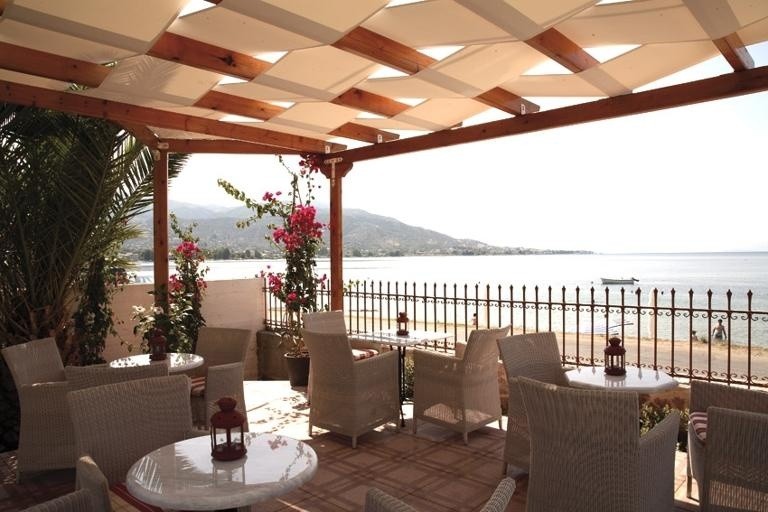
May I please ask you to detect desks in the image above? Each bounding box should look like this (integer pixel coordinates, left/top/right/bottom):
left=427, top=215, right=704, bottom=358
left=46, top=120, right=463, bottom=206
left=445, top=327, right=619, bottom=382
left=348, top=328, right=453, bottom=426
left=126, top=431, right=318, bottom=512
left=565, top=364, right=678, bottom=393
left=110, top=353, right=204, bottom=373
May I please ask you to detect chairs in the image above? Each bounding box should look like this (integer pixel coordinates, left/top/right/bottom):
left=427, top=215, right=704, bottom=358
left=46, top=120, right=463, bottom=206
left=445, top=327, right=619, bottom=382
left=23, top=455, right=114, bottom=512
left=687, top=380, right=768, bottom=512
left=66, top=365, right=168, bottom=388
left=365, top=476, right=516, bottom=512
left=191, top=327, right=251, bottom=430
left=413, top=325, right=512, bottom=445
left=302, top=328, right=399, bottom=448
left=0, top=338, right=109, bottom=486
left=68, top=375, right=195, bottom=512
left=518, top=373, right=681, bottom=512
left=303, top=309, right=378, bottom=407
left=496, top=332, right=567, bottom=473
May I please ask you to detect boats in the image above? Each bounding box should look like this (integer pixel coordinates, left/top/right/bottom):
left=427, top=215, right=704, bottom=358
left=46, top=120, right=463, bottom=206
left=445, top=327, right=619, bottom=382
left=601, top=277, right=633, bottom=286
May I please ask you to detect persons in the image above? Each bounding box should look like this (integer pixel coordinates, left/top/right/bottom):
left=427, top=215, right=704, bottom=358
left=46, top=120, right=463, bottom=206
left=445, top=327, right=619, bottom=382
left=472, top=313, right=476, bottom=326
left=692, top=330, right=699, bottom=341
left=711, top=319, right=727, bottom=341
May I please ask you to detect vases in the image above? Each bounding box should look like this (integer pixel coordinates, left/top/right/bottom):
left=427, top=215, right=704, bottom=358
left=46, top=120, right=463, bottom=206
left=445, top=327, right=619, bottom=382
left=284, top=352, right=310, bottom=387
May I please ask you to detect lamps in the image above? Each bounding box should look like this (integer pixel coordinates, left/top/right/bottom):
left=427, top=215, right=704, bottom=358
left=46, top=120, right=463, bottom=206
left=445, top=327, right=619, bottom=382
left=604, top=337, right=626, bottom=375
left=150, top=330, right=167, bottom=360
left=396, top=312, right=409, bottom=335
left=211, top=397, right=247, bottom=460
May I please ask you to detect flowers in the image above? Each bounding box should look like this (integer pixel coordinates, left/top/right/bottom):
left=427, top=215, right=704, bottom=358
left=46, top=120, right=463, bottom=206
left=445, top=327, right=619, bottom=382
left=130, top=212, right=209, bottom=352
left=216, top=154, right=330, bottom=354
